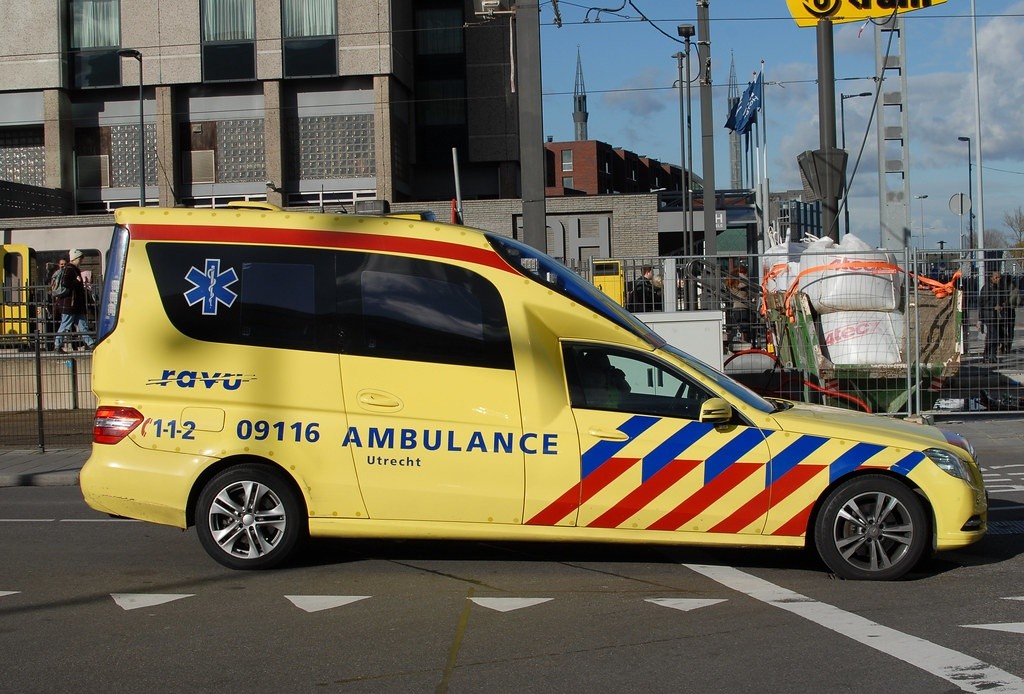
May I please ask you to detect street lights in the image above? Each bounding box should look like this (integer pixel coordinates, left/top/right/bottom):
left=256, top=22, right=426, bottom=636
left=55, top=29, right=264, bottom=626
left=936, top=241, right=946, bottom=260
left=840, top=92, right=872, bottom=235
left=958, top=136, right=973, bottom=291
left=915, top=195, right=928, bottom=249
left=678, top=24, right=696, bottom=255
left=117, top=48, right=146, bottom=207
left=672, top=51, right=687, bottom=254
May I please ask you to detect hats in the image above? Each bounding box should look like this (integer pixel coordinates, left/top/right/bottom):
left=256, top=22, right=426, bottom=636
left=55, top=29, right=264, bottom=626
left=69, top=248, right=83, bottom=262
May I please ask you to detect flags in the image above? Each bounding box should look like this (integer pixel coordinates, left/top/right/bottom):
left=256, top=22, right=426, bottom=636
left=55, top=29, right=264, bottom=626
left=726, top=72, right=760, bottom=137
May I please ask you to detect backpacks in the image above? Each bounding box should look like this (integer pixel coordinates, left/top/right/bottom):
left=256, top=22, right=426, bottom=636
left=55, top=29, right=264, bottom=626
left=51, top=265, right=71, bottom=297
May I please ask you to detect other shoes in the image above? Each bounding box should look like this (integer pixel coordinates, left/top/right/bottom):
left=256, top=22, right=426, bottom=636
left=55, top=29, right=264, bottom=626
left=983, top=355, right=1003, bottom=363
left=1001, top=345, right=1011, bottom=354
left=55, top=348, right=69, bottom=354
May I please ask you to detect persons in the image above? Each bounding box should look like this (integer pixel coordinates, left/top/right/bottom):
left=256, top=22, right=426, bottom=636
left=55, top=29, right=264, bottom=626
left=722, top=265, right=760, bottom=355
left=978, top=271, right=1016, bottom=363
left=676, top=279, right=685, bottom=309
left=52, top=260, right=84, bottom=352
left=626, top=264, right=664, bottom=312
left=52, top=248, right=95, bottom=353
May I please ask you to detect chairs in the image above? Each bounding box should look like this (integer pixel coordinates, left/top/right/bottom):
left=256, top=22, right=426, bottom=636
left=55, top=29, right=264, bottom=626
left=583, top=353, right=617, bottom=410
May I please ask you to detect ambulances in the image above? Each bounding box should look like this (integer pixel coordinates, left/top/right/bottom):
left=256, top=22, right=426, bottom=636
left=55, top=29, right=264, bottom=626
left=79, top=200, right=988, bottom=581
